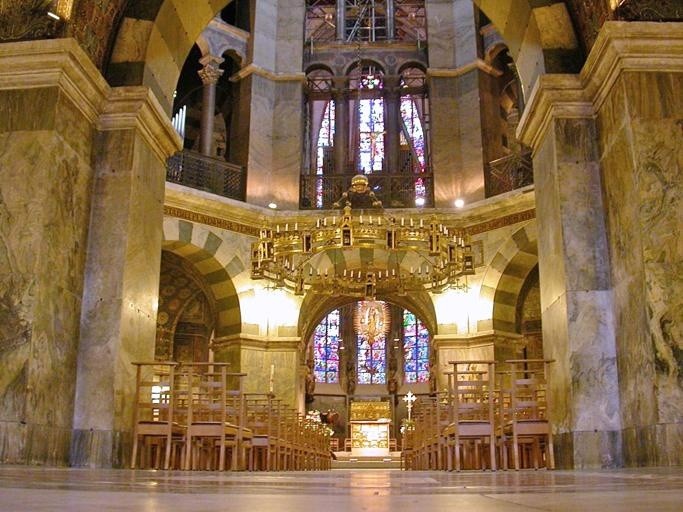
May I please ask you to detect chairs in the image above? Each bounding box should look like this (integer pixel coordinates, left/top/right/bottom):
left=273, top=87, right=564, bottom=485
left=343, top=438, right=351, bottom=452
left=398, top=354, right=558, bottom=471
left=389, top=439, right=397, bottom=451
left=128, top=359, right=339, bottom=473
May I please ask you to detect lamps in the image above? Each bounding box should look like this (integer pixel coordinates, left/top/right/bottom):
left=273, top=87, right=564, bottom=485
left=250, top=1, right=476, bottom=301
